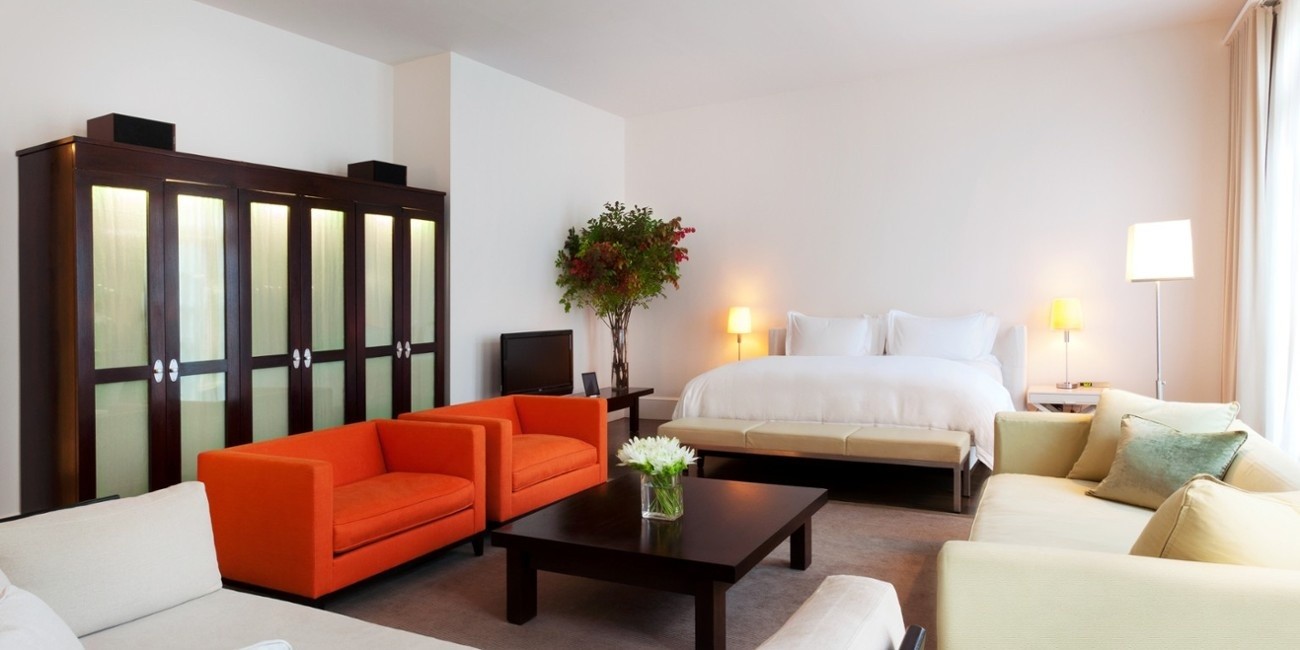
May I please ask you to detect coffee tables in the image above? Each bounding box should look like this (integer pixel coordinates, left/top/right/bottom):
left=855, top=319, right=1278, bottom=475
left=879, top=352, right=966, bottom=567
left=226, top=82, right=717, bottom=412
left=491, top=472, right=829, bottom=650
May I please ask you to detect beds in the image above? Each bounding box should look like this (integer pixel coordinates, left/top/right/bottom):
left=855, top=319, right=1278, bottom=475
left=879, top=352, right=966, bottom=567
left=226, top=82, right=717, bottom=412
left=672, top=310, right=1026, bottom=497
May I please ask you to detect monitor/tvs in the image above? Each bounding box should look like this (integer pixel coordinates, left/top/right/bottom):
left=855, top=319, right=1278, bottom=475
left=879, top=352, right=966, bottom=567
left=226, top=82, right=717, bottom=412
left=499, top=328, right=575, bottom=397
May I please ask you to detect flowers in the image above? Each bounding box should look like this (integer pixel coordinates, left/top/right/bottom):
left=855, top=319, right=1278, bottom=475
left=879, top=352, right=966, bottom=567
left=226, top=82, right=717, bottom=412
left=552, top=196, right=697, bottom=328
left=615, top=434, right=698, bottom=476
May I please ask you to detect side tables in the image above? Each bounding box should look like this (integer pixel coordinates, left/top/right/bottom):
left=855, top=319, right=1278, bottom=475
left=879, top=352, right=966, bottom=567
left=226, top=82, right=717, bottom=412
left=572, top=387, right=654, bottom=445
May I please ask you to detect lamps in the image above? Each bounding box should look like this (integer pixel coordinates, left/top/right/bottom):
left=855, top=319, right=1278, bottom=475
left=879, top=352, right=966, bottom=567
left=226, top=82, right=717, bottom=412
left=726, top=306, right=752, bottom=362
left=1049, top=297, right=1083, bottom=390
left=1126, top=219, right=1196, bottom=401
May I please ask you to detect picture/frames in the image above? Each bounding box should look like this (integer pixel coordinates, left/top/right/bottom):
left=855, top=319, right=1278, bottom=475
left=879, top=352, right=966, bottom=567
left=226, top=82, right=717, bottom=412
left=582, top=372, right=600, bottom=398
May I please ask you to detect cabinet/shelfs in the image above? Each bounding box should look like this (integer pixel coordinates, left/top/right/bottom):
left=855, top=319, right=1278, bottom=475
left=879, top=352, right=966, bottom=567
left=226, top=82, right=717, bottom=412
left=14, top=134, right=446, bottom=518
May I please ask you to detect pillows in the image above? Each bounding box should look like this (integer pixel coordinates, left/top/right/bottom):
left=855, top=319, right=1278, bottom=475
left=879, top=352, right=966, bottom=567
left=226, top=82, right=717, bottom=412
left=0, top=585, right=84, bottom=650
left=785, top=310, right=887, bottom=356
left=1083, top=412, right=1248, bottom=512
left=1066, top=389, right=1242, bottom=484
left=1127, top=472, right=1300, bottom=570
left=885, top=310, right=1000, bottom=361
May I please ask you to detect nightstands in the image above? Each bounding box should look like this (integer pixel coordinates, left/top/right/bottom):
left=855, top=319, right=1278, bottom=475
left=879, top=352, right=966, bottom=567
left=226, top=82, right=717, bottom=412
left=1027, top=384, right=1102, bottom=414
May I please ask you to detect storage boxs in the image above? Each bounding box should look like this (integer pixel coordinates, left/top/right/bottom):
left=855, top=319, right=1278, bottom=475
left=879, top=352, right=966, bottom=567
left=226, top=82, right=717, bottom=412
left=86, top=112, right=177, bottom=151
left=347, top=161, right=408, bottom=185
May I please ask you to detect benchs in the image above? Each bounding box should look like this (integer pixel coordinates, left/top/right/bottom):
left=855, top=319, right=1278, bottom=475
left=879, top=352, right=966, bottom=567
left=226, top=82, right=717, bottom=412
left=658, top=419, right=970, bottom=513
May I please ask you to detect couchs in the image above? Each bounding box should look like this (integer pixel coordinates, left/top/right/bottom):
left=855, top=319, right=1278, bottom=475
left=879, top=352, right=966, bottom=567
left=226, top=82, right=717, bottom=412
left=0, top=477, right=927, bottom=650
left=396, top=394, right=608, bottom=547
left=196, top=419, right=486, bottom=611
left=934, top=407, right=1300, bottom=650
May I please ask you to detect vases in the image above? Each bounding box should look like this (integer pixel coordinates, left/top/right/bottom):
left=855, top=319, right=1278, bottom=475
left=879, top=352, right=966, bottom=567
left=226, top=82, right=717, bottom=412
left=640, top=473, right=684, bottom=521
left=610, top=327, right=629, bottom=397
